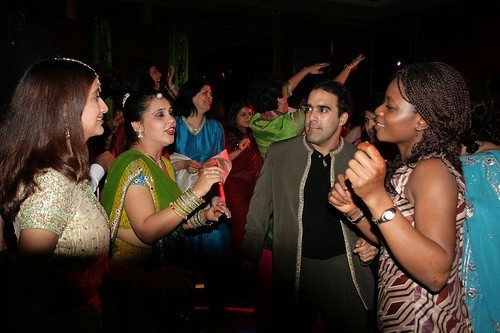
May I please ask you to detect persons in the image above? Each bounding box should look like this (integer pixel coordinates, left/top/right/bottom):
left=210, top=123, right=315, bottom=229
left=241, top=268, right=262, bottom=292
left=101, top=90, right=232, bottom=333
left=0, top=58, right=173, bottom=333
left=224, top=52, right=500, bottom=333
left=171, top=78, right=224, bottom=273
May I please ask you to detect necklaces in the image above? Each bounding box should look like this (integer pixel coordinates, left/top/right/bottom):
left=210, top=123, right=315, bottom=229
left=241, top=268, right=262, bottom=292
left=135, top=139, right=163, bottom=170
left=187, top=116, right=203, bottom=133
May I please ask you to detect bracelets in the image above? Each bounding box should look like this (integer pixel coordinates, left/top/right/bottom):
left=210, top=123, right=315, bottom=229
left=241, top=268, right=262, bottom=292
left=343, top=205, right=361, bottom=217
left=347, top=212, right=367, bottom=225
left=169, top=186, right=212, bottom=230
left=168, top=84, right=175, bottom=90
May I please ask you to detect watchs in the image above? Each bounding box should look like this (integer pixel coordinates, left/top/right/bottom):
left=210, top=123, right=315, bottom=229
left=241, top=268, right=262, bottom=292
left=344, top=64, right=352, bottom=71
left=372, top=202, right=398, bottom=225
